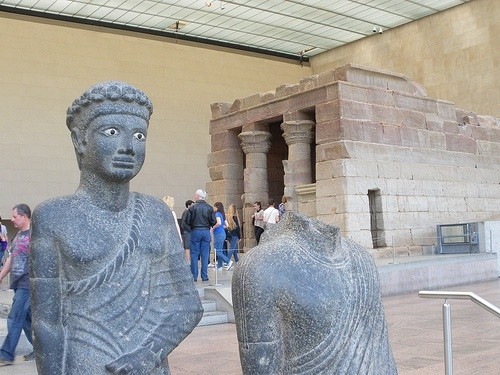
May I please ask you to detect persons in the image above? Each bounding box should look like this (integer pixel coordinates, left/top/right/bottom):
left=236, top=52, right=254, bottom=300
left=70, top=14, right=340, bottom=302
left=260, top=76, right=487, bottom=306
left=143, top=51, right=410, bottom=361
left=230, top=211, right=397, bottom=375
left=251, top=194, right=289, bottom=245
left=0, top=217, right=8, bottom=273
left=29, top=82, right=203, bottom=374
left=0, top=204, right=36, bottom=367
left=161, top=188, right=244, bottom=283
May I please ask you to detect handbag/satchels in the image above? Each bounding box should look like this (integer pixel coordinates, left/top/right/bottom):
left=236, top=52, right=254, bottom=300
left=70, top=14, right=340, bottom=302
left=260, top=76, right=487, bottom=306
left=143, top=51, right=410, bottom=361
left=226, top=231, right=232, bottom=238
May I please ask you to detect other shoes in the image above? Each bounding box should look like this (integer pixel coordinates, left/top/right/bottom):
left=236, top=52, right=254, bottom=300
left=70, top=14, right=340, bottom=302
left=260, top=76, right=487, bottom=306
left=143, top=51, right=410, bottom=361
left=0, top=360, right=13, bottom=366
left=202, top=279, right=208, bottom=281
left=226, top=261, right=233, bottom=270
left=208, top=263, right=215, bottom=267
left=194, top=279, right=197, bottom=282
left=213, top=268, right=222, bottom=271
left=24, top=351, right=34, bottom=359
